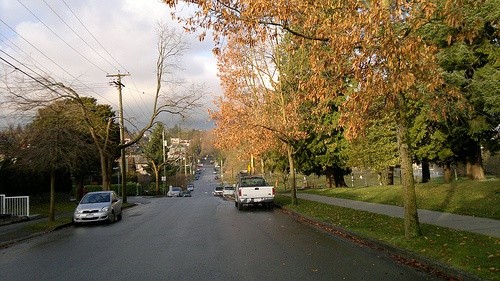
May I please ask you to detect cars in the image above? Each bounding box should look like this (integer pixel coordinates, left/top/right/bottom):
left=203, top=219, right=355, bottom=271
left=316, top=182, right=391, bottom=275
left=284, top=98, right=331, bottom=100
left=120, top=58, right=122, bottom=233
left=74, top=190, right=123, bottom=225
left=222, top=185, right=235, bottom=200
left=214, top=186, right=224, bottom=196
left=168, top=187, right=183, bottom=196
left=186, top=159, right=218, bottom=191
left=184, top=190, right=191, bottom=196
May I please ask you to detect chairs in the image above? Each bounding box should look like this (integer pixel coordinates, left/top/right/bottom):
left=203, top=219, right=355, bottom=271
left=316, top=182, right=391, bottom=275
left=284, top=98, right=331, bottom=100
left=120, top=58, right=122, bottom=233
left=242, top=181, right=248, bottom=186
left=257, top=181, right=261, bottom=185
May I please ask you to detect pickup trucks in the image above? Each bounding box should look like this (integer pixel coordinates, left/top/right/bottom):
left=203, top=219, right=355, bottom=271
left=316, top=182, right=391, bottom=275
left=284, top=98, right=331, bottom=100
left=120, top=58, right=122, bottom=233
left=234, top=175, right=276, bottom=210
